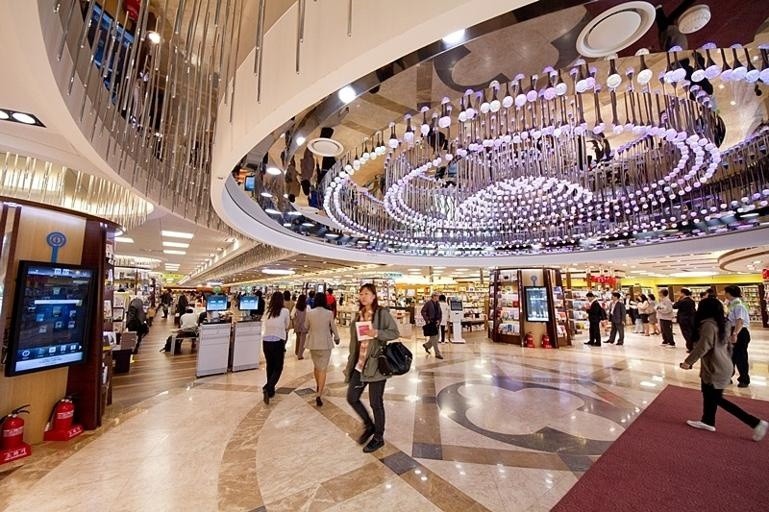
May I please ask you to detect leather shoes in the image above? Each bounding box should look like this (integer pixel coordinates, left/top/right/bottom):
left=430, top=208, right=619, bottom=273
left=603, top=341, right=613, bottom=344
left=738, top=382, right=748, bottom=387
left=263, top=388, right=269, bottom=404
left=436, top=355, right=443, bottom=359
left=616, top=343, right=623, bottom=345
left=268, top=392, right=275, bottom=397
left=423, top=344, right=430, bottom=354
left=591, top=343, right=601, bottom=346
left=358, top=428, right=373, bottom=444
left=364, top=439, right=384, bottom=452
left=584, top=342, right=594, bottom=344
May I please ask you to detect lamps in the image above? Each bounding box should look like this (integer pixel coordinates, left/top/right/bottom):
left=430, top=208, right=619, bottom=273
left=315, top=40, right=769, bottom=258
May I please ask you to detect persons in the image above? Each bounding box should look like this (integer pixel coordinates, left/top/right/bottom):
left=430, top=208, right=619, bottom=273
left=340, top=294, right=345, bottom=306
left=344, top=284, right=400, bottom=453
left=603, top=292, right=626, bottom=346
left=178, top=291, right=187, bottom=328
left=638, top=294, right=651, bottom=336
left=178, top=309, right=198, bottom=353
left=648, top=295, right=660, bottom=335
left=581, top=292, right=602, bottom=346
left=623, top=294, right=634, bottom=325
left=421, top=291, right=444, bottom=359
left=161, top=288, right=172, bottom=319
left=679, top=299, right=768, bottom=442
left=261, top=292, right=290, bottom=404
left=656, top=289, right=676, bottom=347
left=126, top=298, right=148, bottom=336
left=198, top=290, right=206, bottom=303
left=707, top=289, right=716, bottom=296
left=673, top=287, right=699, bottom=353
left=282, top=291, right=296, bottom=352
left=235, top=292, right=243, bottom=308
left=633, top=298, right=645, bottom=334
left=307, top=291, right=317, bottom=309
left=251, top=290, right=264, bottom=320
left=725, top=284, right=751, bottom=388
left=304, top=293, right=340, bottom=406
left=325, top=288, right=337, bottom=337
left=291, top=295, right=311, bottom=361
left=700, top=291, right=708, bottom=302
left=437, top=294, right=451, bottom=346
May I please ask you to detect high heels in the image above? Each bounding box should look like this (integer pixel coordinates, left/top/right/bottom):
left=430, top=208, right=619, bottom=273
left=316, top=397, right=322, bottom=407
left=316, top=387, right=320, bottom=392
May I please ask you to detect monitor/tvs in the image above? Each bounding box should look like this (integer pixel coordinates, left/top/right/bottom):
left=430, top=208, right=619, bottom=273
left=3, top=260, right=98, bottom=377
left=237, top=296, right=260, bottom=311
left=450, top=301, right=462, bottom=311
left=316, top=284, right=324, bottom=293
left=524, top=286, right=550, bottom=322
left=205, top=294, right=227, bottom=312
left=244, top=175, right=255, bottom=191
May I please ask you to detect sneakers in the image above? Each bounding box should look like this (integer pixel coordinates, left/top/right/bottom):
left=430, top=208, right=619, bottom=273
left=665, top=345, right=675, bottom=348
left=687, top=420, right=716, bottom=432
left=752, top=419, right=768, bottom=442
left=659, top=343, right=669, bottom=346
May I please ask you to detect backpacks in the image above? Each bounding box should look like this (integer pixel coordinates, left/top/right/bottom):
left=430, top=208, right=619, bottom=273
left=160, top=335, right=172, bottom=353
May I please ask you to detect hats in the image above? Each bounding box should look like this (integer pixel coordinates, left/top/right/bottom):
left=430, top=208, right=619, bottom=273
left=432, top=291, right=441, bottom=296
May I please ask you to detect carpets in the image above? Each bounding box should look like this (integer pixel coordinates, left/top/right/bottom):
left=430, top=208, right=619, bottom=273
left=548, top=384, right=769, bottom=511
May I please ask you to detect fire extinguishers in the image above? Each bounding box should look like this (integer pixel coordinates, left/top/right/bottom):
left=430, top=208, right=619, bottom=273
left=541, top=332, right=553, bottom=349
left=42, top=395, right=85, bottom=442
left=526, top=330, right=535, bottom=348
left=0, top=404, right=32, bottom=467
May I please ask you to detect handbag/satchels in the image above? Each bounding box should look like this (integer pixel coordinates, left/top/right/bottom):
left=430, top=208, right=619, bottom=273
left=376, top=310, right=413, bottom=376
left=423, top=320, right=437, bottom=336
left=595, top=303, right=607, bottom=320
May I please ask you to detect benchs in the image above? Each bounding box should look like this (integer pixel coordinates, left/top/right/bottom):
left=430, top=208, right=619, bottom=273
left=470, top=320, right=488, bottom=331
left=449, top=321, right=472, bottom=333
left=170, top=336, right=199, bottom=355
